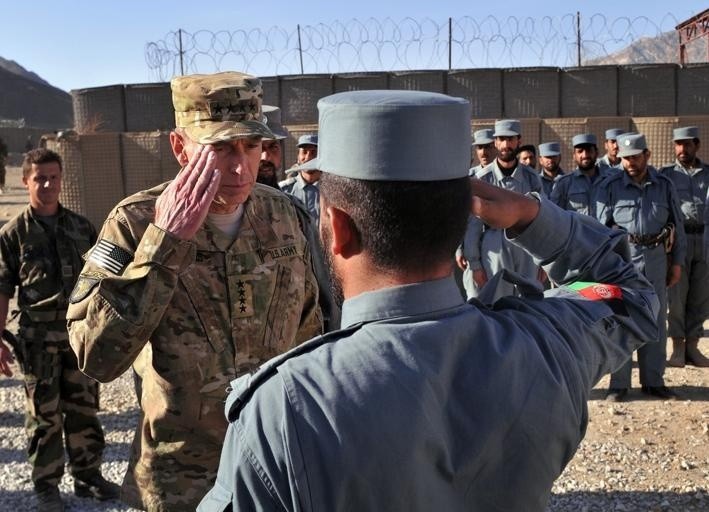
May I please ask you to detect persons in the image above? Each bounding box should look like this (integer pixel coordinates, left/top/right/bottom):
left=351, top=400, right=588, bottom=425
left=66, top=70, right=327, bottom=512
left=193, top=87, right=666, bottom=511
left=0, top=148, right=122, bottom=507
left=259, top=99, right=709, bottom=401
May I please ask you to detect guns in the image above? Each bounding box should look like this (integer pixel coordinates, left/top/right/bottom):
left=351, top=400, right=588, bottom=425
left=2, top=330, right=30, bottom=400
left=657, top=223, right=671, bottom=245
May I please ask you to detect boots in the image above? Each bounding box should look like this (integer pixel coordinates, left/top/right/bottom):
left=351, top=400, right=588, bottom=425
left=34, top=481, right=65, bottom=512
left=74, top=468, right=122, bottom=501
left=667, top=337, right=685, bottom=368
left=685, top=337, right=709, bottom=367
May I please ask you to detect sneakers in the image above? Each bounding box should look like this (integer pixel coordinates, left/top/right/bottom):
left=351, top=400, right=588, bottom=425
left=640, top=384, right=679, bottom=398
left=604, top=388, right=628, bottom=402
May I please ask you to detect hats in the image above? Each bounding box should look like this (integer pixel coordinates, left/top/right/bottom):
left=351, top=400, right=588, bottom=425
left=170, top=71, right=288, bottom=146
left=472, top=118, right=521, bottom=145
left=670, top=126, right=699, bottom=144
left=604, top=127, right=647, bottom=158
left=284, top=90, right=472, bottom=181
left=571, top=133, right=597, bottom=148
left=538, top=141, right=560, bottom=157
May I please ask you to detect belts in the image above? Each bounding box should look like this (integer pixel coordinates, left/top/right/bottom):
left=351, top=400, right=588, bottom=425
left=683, top=223, right=704, bottom=234
left=627, top=232, right=664, bottom=246
